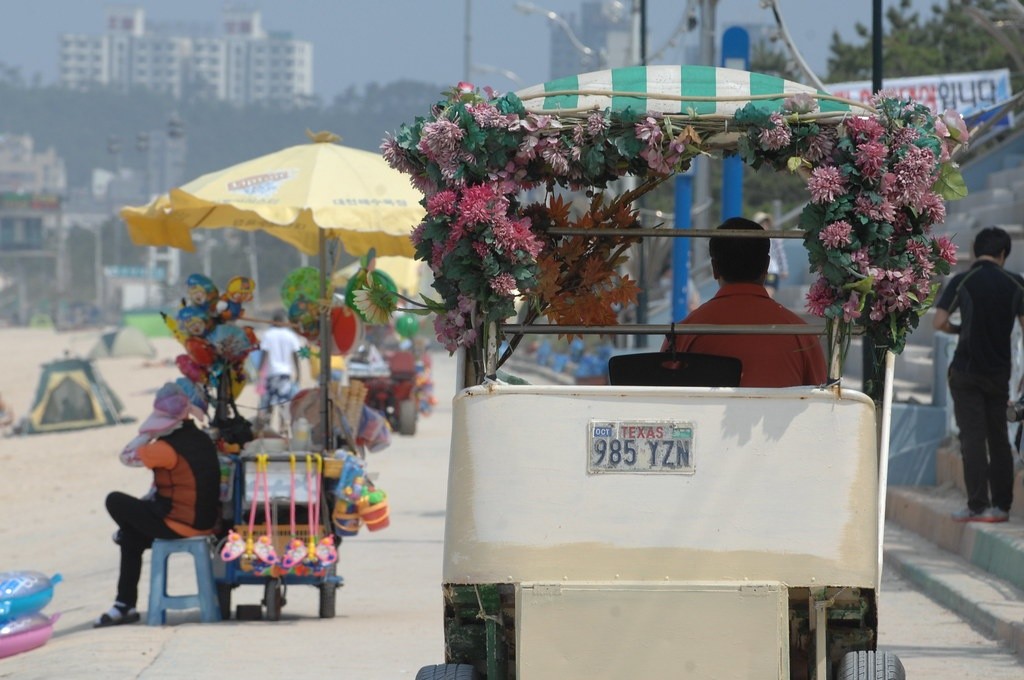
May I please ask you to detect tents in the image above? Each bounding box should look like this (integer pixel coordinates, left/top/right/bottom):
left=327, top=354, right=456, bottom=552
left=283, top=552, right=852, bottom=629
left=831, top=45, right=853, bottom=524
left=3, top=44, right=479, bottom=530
left=7, top=351, right=138, bottom=437
left=86, top=327, right=155, bottom=359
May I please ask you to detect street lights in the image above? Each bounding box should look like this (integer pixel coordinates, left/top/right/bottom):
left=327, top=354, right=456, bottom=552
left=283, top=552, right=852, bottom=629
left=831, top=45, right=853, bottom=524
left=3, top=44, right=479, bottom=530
left=106, top=134, right=124, bottom=322
left=162, top=113, right=185, bottom=291
left=472, top=62, right=526, bottom=88
left=136, top=129, right=156, bottom=308
left=512, top=0, right=598, bottom=58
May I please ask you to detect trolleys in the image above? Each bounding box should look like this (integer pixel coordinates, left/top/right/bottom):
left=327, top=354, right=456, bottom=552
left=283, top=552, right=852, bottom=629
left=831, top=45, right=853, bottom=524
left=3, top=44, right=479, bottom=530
left=219, top=453, right=343, bottom=620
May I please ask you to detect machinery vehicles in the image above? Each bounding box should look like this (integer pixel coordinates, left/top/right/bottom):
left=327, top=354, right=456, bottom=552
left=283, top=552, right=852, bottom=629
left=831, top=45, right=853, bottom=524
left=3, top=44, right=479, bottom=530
left=347, top=351, right=418, bottom=435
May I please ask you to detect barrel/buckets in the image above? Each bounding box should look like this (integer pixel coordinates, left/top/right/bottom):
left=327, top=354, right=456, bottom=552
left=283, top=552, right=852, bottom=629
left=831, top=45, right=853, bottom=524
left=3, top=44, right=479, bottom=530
left=359, top=501, right=390, bottom=532
left=332, top=512, right=359, bottom=536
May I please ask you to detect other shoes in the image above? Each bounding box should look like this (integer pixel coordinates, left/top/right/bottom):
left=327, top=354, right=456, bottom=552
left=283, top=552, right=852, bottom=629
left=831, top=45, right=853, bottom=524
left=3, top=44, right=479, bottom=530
left=953, top=505, right=1008, bottom=523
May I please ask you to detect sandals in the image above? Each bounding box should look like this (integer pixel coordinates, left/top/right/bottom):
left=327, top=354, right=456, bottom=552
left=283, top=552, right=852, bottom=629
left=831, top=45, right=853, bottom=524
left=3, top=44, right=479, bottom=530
left=112, top=530, right=121, bottom=544
left=94, top=604, right=140, bottom=628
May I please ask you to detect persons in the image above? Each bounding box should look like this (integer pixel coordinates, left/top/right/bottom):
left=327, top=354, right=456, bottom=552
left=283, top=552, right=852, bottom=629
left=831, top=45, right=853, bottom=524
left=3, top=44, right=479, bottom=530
left=253, top=308, right=346, bottom=440
left=753, top=213, right=788, bottom=297
left=661, top=265, right=700, bottom=317
left=934, top=225, right=1024, bottom=522
left=659, top=217, right=828, bottom=387
left=92, top=381, right=222, bottom=627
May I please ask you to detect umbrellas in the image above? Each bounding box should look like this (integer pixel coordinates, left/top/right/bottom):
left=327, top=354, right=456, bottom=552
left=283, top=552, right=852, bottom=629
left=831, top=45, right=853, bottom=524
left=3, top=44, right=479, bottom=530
left=333, top=254, right=423, bottom=304
left=123, top=129, right=426, bottom=544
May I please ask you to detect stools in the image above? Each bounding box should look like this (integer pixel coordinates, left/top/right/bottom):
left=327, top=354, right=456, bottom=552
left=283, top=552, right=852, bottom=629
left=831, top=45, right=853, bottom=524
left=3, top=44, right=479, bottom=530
left=144, top=536, right=221, bottom=627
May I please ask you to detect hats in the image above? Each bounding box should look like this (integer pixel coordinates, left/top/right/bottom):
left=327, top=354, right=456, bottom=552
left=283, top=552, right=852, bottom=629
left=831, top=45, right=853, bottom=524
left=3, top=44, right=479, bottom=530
left=138, top=381, right=204, bottom=432
